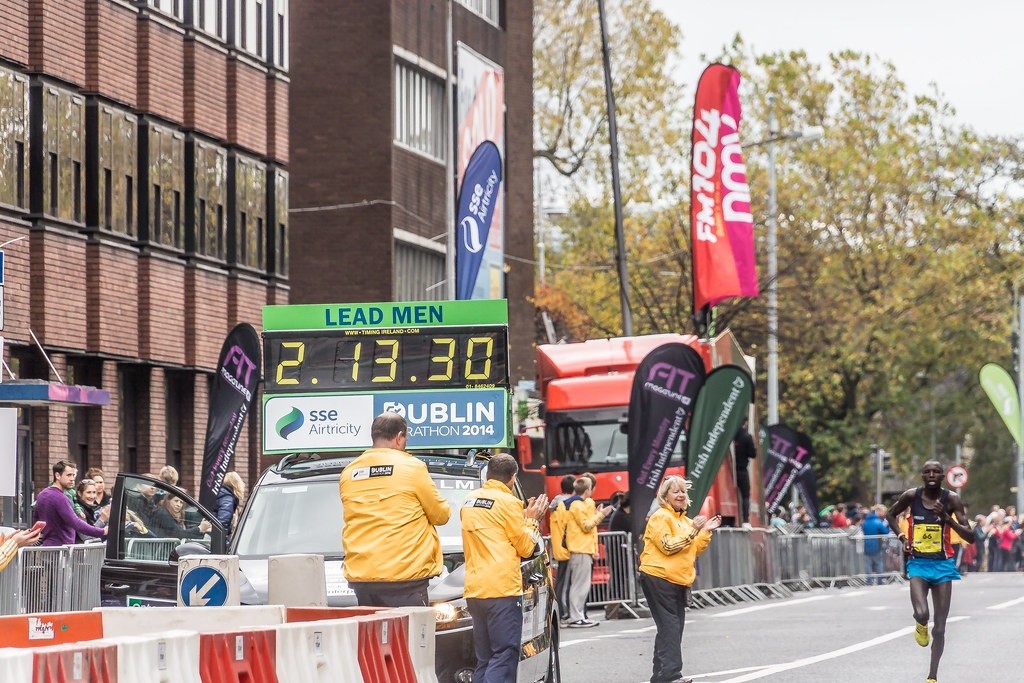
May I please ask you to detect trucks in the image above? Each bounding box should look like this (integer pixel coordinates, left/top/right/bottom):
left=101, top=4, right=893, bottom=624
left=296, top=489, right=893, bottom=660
left=517, top=329, right=771, bottom=595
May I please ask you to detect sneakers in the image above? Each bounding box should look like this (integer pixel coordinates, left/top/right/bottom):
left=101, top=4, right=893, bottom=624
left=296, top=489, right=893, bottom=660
left=914, top=621, right=930, bottom=647
left=925, top=675, right=937, bottom=683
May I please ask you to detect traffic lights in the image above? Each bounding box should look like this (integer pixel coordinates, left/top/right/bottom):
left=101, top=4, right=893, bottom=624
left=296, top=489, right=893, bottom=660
left=883, top=453, right=891, bottom=471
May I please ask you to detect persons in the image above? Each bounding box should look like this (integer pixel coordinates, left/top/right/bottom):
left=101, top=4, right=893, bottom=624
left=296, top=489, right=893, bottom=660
left=639, top=476, right=722, bottom=683
left=549, top=472, right=613, bottom=628
left=861, top=507, right=890, bottom=586
left=460, top=453, right=549, bottom=683
left=950, top=503, right=1024, bottom=576
left=885, top=458, right=975, bottom=683
left=607, top=491, right=631, bottom=607
left=64, top=467, right=157, bottom=612
left=770, top=502, right=911, bottom=571
left=339, top=411, right=451, bottom=607
left=127, top=465, right=212, bottom=539
left=0, top=528, right=41, bottom=571
left=26, top=460, right=108, bottom=614
left=211, top=471, right=245, bottom=535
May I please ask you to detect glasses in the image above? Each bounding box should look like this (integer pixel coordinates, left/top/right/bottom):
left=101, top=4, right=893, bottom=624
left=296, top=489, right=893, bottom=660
left=78, top=479, right=94, bottom=490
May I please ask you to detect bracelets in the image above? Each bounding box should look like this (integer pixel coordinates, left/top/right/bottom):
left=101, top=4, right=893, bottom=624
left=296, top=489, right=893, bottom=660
left=897, top=532, right=905, bottom=540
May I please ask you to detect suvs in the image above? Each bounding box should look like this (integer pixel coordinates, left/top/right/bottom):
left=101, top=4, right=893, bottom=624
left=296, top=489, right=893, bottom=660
left=97, top=454, right=562, bottom=683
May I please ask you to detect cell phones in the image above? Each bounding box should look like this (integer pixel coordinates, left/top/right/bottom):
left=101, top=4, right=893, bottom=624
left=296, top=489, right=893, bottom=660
left=28, top=522, right=47, bottom=536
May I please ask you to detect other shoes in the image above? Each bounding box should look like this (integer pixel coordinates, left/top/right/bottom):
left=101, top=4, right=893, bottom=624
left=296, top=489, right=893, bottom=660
left=669, top=678, right=692, bottom=683
left=560, top=618, right=599, bottom=628
